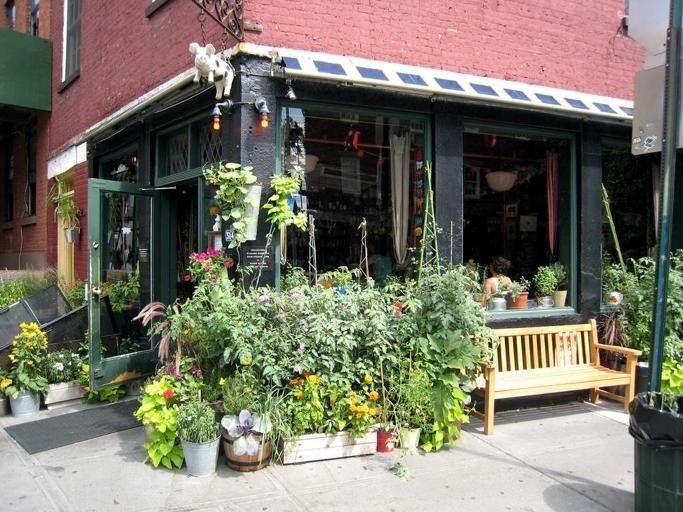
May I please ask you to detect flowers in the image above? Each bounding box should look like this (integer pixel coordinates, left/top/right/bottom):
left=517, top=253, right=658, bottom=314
left=90, top=278, right=139, bottom=314
left=131, top=349, right=385, bottom=469
left=181, top=244, right=235, bottom=299
left=0, top=321, right=129, bottom=403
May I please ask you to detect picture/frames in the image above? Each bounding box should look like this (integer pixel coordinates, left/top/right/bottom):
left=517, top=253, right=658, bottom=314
left=503, top=200, right=540, bottom=234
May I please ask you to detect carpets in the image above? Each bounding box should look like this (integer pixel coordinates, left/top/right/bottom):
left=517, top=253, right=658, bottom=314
left=1, top=399, right=143, bottom=455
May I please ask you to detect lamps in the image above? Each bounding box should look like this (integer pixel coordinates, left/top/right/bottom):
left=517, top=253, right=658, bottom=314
left=211, top=97, right=271, bottom=131
left=485, top=140, right=519, bottom=192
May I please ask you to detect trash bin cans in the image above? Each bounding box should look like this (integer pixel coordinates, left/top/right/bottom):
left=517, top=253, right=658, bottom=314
left=628, top=391, right=683, bottom=512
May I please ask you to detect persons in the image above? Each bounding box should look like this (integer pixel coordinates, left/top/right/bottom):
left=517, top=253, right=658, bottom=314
left=478, top=255, right=515, bottom=308
left=355, top=238, right=392, bottom=282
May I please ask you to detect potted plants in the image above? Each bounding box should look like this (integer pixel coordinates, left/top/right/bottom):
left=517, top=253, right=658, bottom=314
left=201, top=159, right=308, bottom=249
left=46, top=173, right=81, bottom=243
left=395, top=381, right=435, bottom=451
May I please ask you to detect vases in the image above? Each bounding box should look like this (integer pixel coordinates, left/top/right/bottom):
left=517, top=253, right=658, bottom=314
left=179, top=421, right=401, bottom=473
left=0, top=379, right=94, bottom=417
left=109, top=289, right=132, bottom=309
left=490, top=261, right=569, bottom=311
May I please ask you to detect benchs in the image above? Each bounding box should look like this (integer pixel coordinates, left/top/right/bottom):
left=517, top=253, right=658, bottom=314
left=457, top=317, right=643, bottom=435
